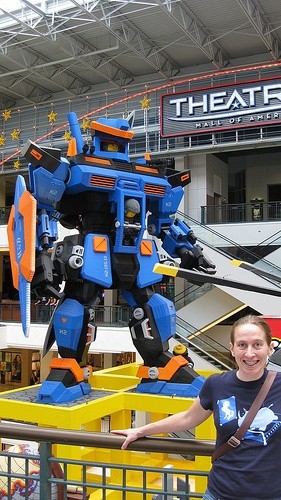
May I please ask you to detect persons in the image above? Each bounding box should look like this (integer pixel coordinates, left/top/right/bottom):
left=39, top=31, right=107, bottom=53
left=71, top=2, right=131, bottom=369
left=34, top=296, right=59, bottom=322
left=12, top=355, right=20, bottom=379
left=107, top=315, right=281, bottom=500
